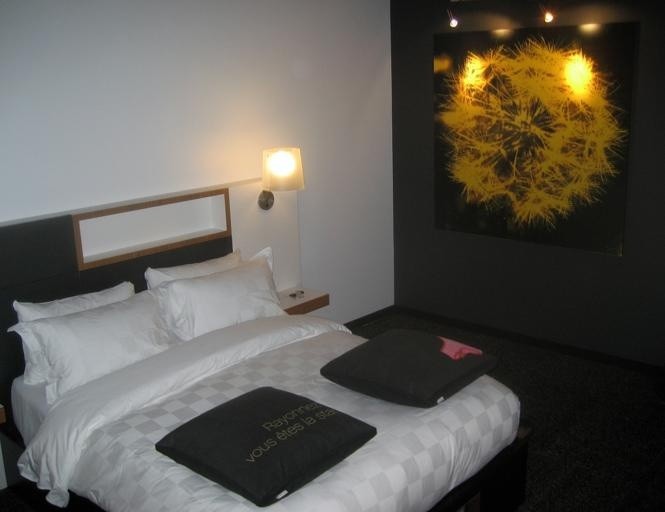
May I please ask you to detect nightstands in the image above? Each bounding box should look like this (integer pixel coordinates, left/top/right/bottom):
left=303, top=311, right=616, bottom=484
left=277, top=285, right=330, bottom=319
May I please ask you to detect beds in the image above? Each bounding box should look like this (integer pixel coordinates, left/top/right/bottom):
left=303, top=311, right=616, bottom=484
left=0, top=186, right=538, bottom=512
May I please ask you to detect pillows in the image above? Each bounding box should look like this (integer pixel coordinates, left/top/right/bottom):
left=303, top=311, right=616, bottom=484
left=144, top=250, right=241, bottom=290
left=155, top=386, right=378, bottom=507
left=13, top=281, right=136, bottom=385
left=159, top=247, right=286, bottom=342
left=320, top=326, right=499, bottom=410
left=10, top=292, right=172, bottom=405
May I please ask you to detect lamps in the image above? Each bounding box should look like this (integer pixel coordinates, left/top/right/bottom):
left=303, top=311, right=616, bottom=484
left=258, top=146, right=306, bottom=212
left=538, top=2, right=557, bottom=24
left=443, top=7, right=461, bottom=27
